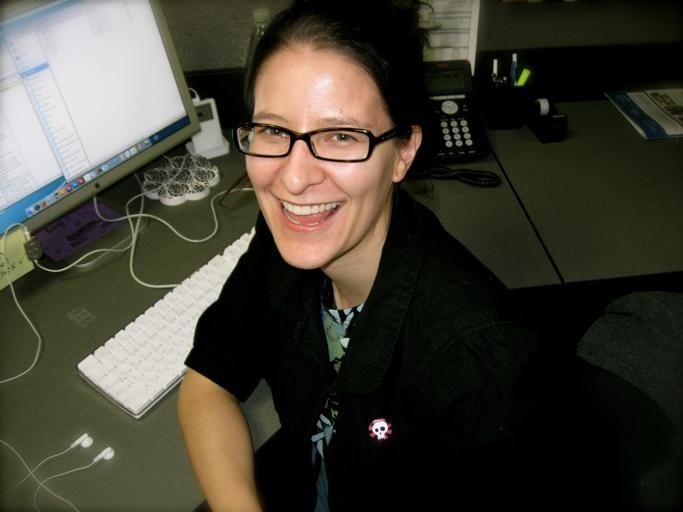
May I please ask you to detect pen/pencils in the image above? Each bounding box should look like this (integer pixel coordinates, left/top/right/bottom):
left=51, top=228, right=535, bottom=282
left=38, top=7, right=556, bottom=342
left=491, top=53, right=531, bottom=87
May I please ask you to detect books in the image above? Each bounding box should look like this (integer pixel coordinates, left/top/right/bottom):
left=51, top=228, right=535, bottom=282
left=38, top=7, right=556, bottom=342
left=603, top=80, right=683, bottom=148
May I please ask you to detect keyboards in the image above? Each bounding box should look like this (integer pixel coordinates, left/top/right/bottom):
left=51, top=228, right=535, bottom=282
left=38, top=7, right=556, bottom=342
left=75, top=225, right=261, bottom=422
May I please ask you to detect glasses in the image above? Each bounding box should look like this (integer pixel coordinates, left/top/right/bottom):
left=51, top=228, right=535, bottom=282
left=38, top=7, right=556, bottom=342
left=233, top=123, right=395, bottom=162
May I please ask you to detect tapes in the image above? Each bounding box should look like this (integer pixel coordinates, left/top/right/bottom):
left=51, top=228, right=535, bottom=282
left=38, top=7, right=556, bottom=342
left=535, top=98, right=549, bottom=116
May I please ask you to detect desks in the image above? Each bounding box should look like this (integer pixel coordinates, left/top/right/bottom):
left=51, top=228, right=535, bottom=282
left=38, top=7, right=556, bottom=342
left=0, top=66, right=280, bottom=511
left=399, top=43, right=682, bottom=317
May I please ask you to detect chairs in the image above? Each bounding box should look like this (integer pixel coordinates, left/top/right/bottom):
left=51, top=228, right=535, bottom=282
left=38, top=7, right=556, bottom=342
left=577, top=291, right=683, bottom=423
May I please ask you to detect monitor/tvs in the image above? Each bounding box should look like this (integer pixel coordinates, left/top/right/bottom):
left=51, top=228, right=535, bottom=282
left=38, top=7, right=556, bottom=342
left=1, top=0, right=202, bottom=270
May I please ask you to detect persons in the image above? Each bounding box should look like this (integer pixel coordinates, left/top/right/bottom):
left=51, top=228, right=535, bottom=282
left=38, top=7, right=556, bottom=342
left=173, top=1, right=582, bottom=512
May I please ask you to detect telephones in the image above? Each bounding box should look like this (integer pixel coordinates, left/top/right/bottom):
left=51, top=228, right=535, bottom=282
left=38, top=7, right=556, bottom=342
left=415, top=59, right=492, bottom=167
